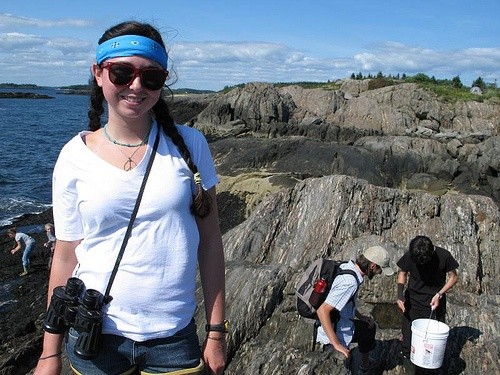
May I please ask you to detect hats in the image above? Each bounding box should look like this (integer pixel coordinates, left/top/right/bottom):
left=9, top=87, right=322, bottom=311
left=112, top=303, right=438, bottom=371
left=363, top=246, right=394, bottom=275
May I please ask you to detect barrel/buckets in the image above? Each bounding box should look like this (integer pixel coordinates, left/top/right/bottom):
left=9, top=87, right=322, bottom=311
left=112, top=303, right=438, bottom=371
left=409, top=308, right=450, bottom=370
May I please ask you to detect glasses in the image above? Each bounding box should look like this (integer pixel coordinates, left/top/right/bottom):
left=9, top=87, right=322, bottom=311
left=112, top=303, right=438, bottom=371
left=100, top=62, right=169, bottom=91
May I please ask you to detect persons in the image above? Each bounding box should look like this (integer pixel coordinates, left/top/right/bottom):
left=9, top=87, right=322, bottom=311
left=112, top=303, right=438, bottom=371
left=314, top=247, right=394, bottom=375
left=397, top=235, right=458, bottom=375
left=33, top=20, right=228, bottom=375
left=8, top=228, right=36, bottom=277
left=43, top=222, right=56, bottom=268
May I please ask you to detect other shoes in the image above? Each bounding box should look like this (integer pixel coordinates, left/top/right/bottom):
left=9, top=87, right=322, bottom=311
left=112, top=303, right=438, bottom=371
left=358, top=357, right=377, bottom=375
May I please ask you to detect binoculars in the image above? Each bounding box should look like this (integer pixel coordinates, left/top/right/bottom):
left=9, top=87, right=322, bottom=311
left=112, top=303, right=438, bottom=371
left=42, top=277, right=104, bottom=360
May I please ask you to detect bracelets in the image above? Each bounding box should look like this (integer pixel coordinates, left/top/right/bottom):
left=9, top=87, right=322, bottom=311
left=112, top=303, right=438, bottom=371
left=436, top=293, right=442, bottom=299
left=39, top=351, right=62, bottom=360
left=207, top=334, right=226, bottom=340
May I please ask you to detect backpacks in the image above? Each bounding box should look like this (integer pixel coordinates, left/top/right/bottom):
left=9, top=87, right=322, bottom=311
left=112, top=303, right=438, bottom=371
left=296, top=258, right=360, bottom=320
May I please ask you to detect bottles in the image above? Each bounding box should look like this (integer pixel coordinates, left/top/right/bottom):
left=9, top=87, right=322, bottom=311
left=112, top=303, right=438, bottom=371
left=310, top=275, right=329, bottom=307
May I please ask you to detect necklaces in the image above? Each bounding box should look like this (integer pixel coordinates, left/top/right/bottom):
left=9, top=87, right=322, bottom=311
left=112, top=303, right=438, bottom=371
left=115, top=128, right=149, bottom=171
left=103, top=123, right=152, bottom=147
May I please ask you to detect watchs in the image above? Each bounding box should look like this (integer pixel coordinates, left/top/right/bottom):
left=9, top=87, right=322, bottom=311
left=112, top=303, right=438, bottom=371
left=205, top=319, right=229, bottom=333
